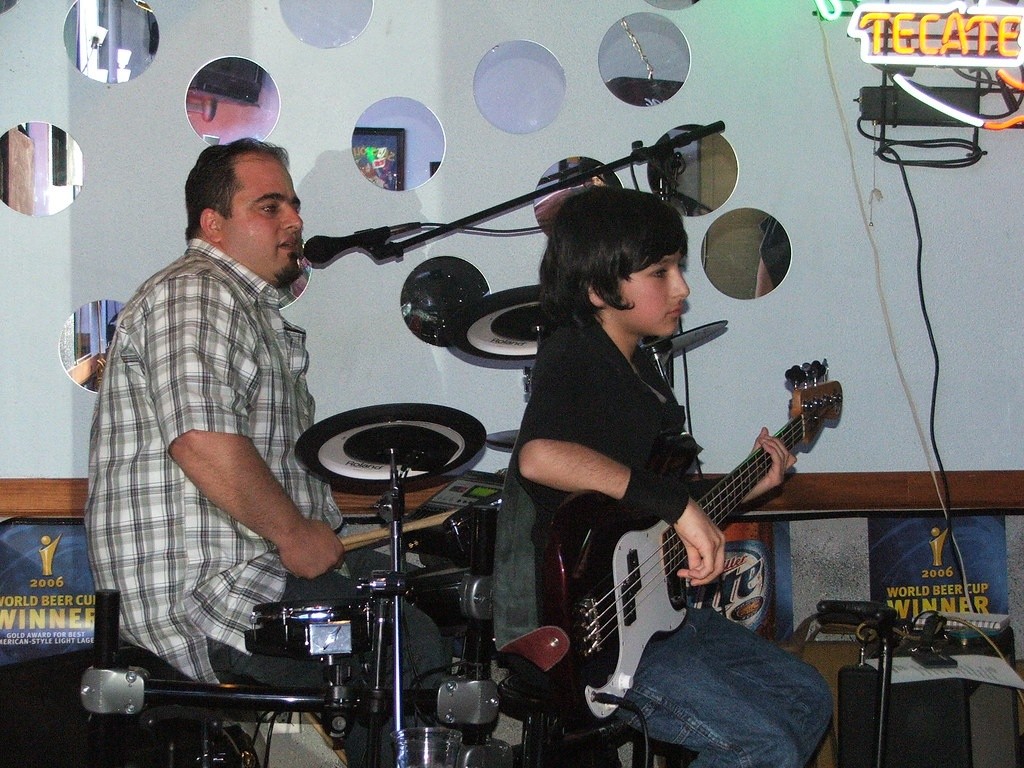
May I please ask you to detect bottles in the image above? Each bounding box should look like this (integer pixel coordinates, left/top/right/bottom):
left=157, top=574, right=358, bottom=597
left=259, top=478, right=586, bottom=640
left=389, top=726, right=463, bottom=768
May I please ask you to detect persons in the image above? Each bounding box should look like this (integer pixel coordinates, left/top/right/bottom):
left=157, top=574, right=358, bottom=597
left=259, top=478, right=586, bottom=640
left=517, top=187, right=834, bottom=768
left=756, top=216, right=791, bottom=298
left=81, top=136, right=445, bottom=768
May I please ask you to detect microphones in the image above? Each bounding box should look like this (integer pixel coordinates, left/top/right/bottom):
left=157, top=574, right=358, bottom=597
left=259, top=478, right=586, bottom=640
left=304, top=221, right=422, bottom=264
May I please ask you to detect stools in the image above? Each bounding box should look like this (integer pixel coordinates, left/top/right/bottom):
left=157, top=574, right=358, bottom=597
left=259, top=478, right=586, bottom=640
left=498, top=676, right=626, bottom=768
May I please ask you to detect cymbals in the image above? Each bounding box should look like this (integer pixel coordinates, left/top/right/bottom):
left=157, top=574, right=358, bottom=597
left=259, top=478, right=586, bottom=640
left=639, top=318, right=728, bottom=358
left=447, top=284, right=555, bottom=360
left=483, top=429, right=519, bottom=448
left=294, top=402, right=487, bottom=487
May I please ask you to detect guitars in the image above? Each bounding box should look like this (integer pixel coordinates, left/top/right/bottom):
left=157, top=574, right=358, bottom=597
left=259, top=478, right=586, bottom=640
left=544, top=359, right=844, bottom=721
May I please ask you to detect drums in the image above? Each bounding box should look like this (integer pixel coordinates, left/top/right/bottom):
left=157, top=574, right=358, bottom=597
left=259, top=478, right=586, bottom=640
left=443, top=489, right=504, bottom=682
left=251, top=593, right=384, bottom=658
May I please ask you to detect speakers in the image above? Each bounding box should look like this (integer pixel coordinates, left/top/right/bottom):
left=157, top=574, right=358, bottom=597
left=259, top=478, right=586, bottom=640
left=802, top=627, right=1024, bottom=768
